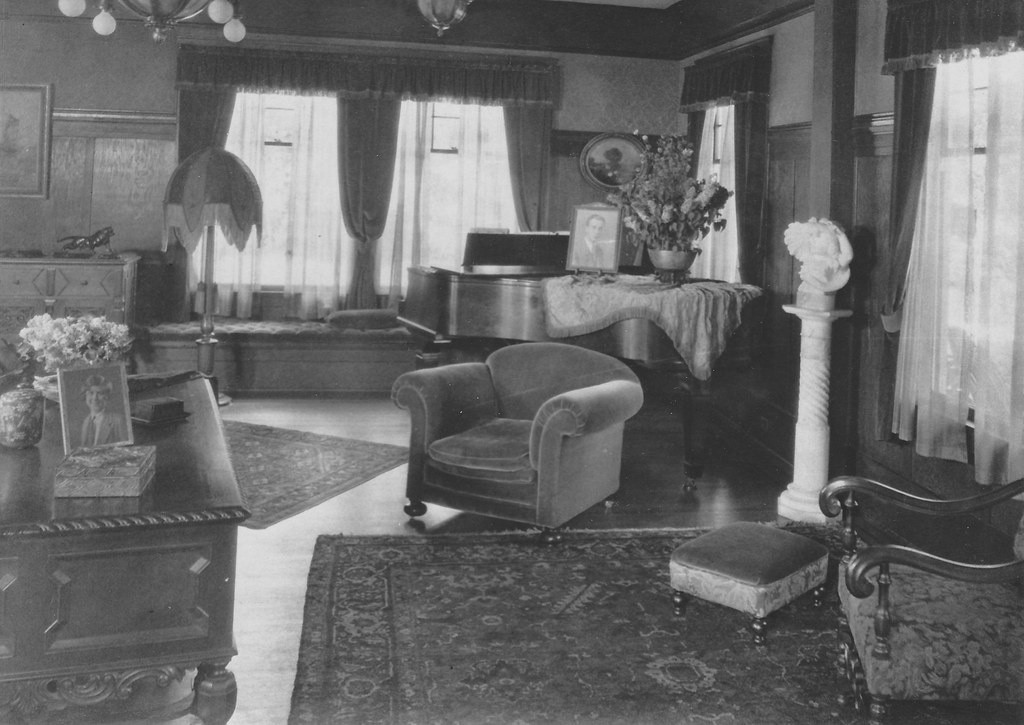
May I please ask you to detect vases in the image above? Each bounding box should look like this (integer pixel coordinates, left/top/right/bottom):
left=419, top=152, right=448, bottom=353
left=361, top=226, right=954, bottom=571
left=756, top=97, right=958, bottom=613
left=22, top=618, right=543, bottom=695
left=647, top=249, right=698, bottom=286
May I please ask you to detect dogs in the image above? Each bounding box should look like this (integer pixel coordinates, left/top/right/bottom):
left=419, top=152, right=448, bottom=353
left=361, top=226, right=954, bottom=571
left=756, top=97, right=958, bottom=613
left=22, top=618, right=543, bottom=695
left=57, top=226, right=116, bottom=254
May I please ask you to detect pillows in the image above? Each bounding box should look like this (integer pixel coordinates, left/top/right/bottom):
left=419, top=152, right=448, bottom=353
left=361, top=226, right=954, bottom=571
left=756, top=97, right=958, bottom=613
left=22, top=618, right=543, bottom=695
left=322, top=309, right=399, bottom=330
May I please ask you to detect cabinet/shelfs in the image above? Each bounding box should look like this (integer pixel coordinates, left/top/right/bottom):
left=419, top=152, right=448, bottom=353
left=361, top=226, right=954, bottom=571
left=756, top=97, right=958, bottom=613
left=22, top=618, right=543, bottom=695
left=1, top=252, right=141, bottom=367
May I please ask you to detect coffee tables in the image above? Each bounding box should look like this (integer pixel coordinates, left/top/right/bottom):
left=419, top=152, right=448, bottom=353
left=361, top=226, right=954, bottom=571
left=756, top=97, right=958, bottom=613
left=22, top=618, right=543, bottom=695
left=0, top=371, right=251, bottom=725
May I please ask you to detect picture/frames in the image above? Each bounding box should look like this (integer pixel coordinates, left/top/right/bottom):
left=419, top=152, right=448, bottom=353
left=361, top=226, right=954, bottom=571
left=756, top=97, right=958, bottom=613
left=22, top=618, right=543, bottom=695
left=42, top=107, right=180, bottom=265
left=565, top=204, right=624, bottom=275
left=57, top=361, right=133, bottom=456
left=580, top=132, right=652, bottom=195
left=0, top=84, right=54, bottom=199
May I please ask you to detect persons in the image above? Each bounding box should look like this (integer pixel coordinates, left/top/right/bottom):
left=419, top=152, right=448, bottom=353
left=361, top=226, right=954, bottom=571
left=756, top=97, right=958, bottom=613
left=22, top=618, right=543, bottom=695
left=79, top=375, right=127, bottom=446
left=588, top=149, right=625, bottom=185
left=574, top=214, right=608, bottom=267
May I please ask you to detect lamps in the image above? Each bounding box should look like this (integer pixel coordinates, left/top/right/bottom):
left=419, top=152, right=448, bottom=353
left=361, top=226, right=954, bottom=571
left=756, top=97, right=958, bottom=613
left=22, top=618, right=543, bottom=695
left=159, top=145, right=267, bottom=409
left=415, top=0, right=473, bottom=37
left=57, top=0, right=247, bottom=46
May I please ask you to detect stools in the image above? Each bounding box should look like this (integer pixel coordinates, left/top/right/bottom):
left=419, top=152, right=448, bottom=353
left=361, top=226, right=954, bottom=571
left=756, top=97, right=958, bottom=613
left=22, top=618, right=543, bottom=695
left=669, top=521, right=830, bottom=645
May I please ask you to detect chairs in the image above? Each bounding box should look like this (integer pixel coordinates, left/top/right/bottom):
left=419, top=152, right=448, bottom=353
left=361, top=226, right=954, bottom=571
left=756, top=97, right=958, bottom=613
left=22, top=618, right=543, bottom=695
left=816, top=474, right=1024, bottom=725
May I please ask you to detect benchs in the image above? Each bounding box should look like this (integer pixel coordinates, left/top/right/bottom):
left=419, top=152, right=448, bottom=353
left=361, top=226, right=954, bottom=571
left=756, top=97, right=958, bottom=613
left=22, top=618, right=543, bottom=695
left=143, top=318, right=475, bottom=395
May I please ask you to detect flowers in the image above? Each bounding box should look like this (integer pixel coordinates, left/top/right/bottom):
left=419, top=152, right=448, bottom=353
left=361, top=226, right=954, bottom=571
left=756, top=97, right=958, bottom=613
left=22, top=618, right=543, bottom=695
left=15, top=311, right=134, bottom=372
left=605, top=128, right=735, bottom=256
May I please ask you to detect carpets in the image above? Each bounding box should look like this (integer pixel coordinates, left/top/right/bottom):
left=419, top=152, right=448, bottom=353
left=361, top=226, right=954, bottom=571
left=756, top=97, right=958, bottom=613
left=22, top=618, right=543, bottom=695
left=286, top=520, right=1024, bottom=725
left=221, top=418, right=410, bottom=530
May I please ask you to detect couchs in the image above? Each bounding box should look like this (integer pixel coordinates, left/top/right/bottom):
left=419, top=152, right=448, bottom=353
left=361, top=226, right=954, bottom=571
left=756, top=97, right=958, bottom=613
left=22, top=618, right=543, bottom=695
left=390, top=343, right=645, bottom=550
